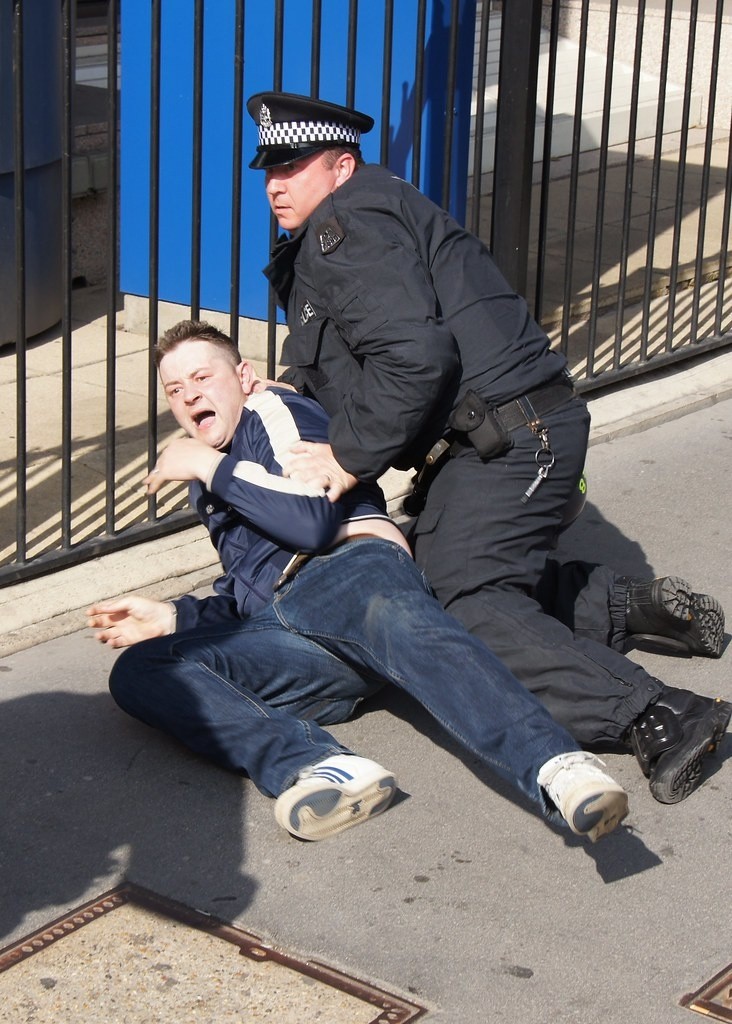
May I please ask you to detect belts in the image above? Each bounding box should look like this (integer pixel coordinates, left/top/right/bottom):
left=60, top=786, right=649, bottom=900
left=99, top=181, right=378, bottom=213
left=410, top=374, right=584, bottom=466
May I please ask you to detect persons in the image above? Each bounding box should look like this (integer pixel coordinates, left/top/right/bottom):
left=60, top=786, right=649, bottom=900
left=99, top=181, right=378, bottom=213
left=244, top=91, right=732, bottom=805
left=85, top=320, right=631, bottom=845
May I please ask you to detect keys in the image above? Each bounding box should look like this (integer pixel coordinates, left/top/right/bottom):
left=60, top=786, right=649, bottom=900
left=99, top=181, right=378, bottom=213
left=520, top=466, right=551, bottom=504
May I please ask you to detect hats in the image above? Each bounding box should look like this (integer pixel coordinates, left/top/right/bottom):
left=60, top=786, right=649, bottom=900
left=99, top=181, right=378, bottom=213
left=241, top=92, right=376, bottom=174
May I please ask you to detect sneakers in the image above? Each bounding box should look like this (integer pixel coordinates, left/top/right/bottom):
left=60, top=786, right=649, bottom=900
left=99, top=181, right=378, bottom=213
left=274, top=751, right=398, bottom=842
left=619, top=576, right=727, bottom=661
left=617, top=677, right=728, bottom=804
left=528, top=745, right=635, bottom=849
